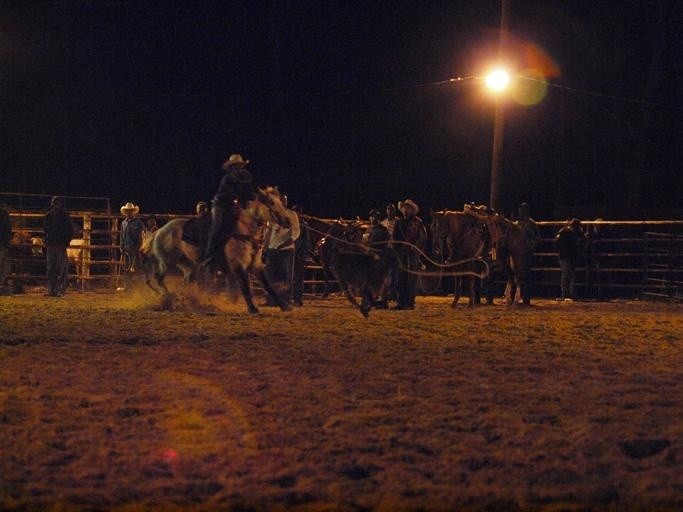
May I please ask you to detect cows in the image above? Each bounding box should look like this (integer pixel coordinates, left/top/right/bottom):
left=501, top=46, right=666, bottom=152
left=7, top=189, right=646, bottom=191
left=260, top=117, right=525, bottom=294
left=329, top=247, right=404, bottom=319
left=29, top=235, right=84, bottom=288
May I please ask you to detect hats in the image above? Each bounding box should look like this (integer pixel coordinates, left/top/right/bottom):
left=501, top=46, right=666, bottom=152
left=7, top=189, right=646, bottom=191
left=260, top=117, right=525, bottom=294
left=223, top=153, right=250, bottom=170
left=120, top=201, right=140, bottom=217
left=397, top=198, right=420, bottom=216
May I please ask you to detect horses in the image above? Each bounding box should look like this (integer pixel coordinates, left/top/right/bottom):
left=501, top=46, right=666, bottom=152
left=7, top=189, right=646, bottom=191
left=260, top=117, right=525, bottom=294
left=429, top=207, right=523, bottom=307
left=294, top=208, right=390, bottom=310
left=140, top=185, right=292, bottom=315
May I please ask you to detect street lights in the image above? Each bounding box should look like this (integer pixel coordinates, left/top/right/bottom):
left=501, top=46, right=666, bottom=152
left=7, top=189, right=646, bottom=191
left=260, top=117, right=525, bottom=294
left=482, top=68, right=509, bottom=210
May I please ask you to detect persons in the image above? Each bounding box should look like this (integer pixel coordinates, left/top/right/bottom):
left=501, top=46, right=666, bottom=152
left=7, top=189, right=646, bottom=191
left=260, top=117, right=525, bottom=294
left=42, top=196, right=74, bottom=298
left=0, top=198, right=14, bottom=295
